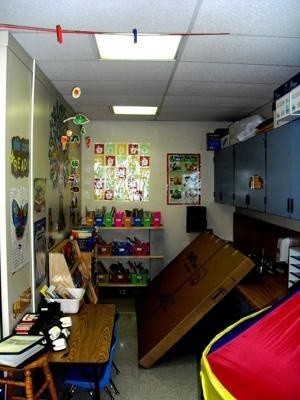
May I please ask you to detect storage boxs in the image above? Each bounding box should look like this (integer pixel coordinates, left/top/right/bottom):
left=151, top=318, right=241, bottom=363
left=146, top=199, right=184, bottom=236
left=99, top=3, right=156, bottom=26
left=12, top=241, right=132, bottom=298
left=275, top=91, right=296, bottom=122
left=289, top=84, right=300, bottom=117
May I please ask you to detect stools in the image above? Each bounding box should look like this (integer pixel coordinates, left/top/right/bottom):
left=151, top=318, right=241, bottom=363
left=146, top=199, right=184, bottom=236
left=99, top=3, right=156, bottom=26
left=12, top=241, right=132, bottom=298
left=0, top=356, right=59, bottom=400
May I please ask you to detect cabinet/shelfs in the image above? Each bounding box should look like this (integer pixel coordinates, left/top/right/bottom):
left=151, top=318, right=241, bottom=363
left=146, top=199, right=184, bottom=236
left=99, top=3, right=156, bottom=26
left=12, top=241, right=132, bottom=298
left=233, top=133, right=266, bottom=214
left=49, top=237, right=98, bottom=306
left=212, top=145, right=233, bottom=207
left=265, top=118, right=300, bottom=222
left=70, top=225, right=165, bottom=299
left=287, top=246, right=300, bottom=291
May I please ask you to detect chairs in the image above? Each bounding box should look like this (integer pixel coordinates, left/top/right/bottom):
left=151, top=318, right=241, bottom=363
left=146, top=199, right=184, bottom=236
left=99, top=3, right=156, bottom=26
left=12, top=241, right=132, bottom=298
left=59, top=311, right=120, bottom=400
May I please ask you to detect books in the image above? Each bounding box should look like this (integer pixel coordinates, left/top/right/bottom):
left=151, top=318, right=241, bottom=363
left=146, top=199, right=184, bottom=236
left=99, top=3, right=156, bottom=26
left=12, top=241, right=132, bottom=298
left=0, top=333, right=51, bottom=369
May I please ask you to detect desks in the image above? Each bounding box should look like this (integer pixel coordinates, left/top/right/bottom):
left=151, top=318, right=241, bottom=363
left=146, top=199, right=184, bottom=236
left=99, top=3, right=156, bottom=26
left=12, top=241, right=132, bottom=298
left=42, top=302, right=116, bottom=400
left=233, top=273, right=287, bottom=322
